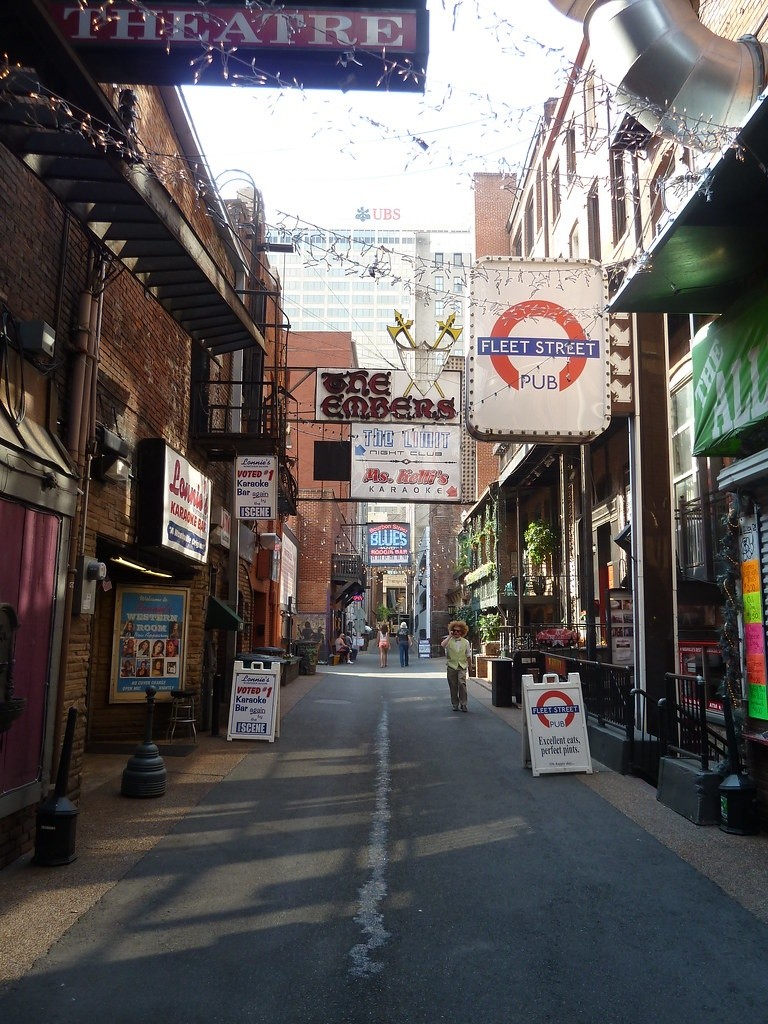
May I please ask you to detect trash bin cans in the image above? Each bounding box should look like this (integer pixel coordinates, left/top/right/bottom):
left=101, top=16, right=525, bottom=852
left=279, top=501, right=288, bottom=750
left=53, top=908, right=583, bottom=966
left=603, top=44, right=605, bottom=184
left=233, top=647, right=285, bottom=674
left=350, top=628, right=379, bottom=660
left=295, top=641, right=321, bottom=675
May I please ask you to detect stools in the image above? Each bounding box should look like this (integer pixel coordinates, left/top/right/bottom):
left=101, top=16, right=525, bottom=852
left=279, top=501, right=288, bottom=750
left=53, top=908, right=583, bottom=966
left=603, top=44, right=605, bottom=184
left=165, top=689, right=199, bottom=744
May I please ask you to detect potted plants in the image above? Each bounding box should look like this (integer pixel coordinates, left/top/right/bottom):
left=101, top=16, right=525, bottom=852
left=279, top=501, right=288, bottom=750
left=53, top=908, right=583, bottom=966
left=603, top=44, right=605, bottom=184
left=469, top=522, right=494, bottom=550
left=478, top=614, right=501, bottom=656
left=527, top=522, right=559, bottom=595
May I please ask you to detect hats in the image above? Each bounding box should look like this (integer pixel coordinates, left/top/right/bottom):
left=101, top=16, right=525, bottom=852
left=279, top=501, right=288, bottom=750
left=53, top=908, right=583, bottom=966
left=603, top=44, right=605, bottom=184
left=400, top=622, right=407, bottom=629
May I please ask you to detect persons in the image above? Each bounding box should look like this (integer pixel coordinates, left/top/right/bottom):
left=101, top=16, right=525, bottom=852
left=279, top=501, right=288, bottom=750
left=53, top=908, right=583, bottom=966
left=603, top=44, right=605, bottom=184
left=376, top=624, right=391, bottom=667
left=335, top=633, right=358, bottom=664
left=396, top=622, right=412, bottom=667
left=120, top=622, right=179, bottom=678
left=442, top=621, right=471, bottom=711
left=365, top=622, right=372, bottom=633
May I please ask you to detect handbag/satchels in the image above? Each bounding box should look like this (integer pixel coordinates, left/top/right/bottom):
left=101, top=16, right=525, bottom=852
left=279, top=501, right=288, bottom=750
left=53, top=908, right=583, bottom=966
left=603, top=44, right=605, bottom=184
left=339, top=648, right=346, bottom=652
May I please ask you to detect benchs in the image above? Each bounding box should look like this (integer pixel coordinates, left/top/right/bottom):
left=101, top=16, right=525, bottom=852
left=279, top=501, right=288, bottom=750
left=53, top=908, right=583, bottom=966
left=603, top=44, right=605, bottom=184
left=329, top=639, right=347, bottom=665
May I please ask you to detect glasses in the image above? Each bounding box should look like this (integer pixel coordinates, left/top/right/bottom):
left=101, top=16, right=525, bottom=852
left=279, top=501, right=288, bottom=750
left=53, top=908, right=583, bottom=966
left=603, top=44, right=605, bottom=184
left=453, top=630, right=461, bottom=633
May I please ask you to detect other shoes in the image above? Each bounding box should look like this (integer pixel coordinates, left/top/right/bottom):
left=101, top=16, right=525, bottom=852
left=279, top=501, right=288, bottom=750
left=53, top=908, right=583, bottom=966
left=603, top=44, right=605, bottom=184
left=452, top=706, right=458, bottom=711
left=347, top=660, right=354, bottom=664
left=461, top=705, right=468, bottom=712
